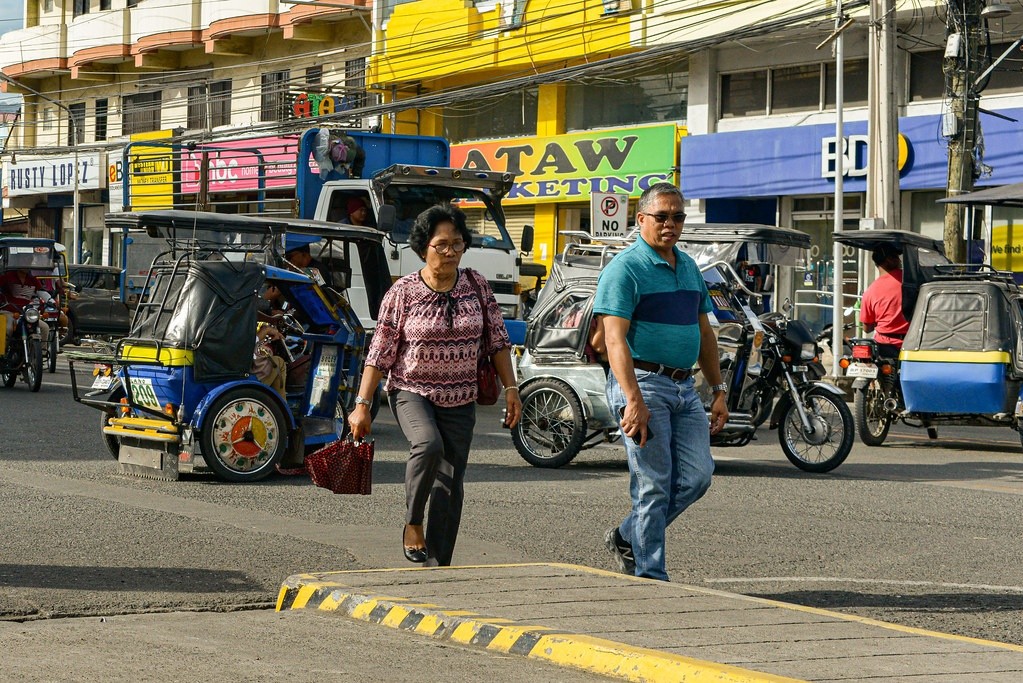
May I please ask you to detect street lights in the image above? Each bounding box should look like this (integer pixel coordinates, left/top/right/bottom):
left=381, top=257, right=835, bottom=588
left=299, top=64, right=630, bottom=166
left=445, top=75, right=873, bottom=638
left=0, top=72, right=80, bottom=265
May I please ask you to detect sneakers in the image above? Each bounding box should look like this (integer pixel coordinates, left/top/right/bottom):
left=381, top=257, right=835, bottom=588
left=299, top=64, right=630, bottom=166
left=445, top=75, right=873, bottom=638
left=603, top=527, right=636, bottom=576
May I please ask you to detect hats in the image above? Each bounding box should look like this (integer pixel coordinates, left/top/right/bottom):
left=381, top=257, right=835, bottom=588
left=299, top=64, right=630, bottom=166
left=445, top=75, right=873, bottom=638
left=345, top=197, right=366, bottom=214
left=872, top=242, right=903, bottom=266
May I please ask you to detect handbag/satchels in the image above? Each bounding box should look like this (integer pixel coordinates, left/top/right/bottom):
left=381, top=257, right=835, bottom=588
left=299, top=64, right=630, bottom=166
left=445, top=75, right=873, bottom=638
left=477, top=356, right=503, bottom=405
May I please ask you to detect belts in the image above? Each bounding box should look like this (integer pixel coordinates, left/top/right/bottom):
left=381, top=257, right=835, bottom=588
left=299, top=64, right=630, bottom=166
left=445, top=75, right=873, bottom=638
left=633, top=358, right=702, bottom=380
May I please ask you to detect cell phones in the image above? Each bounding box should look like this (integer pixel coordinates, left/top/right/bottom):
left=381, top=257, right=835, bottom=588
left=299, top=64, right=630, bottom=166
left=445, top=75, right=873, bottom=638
left=619, top=405, right=654, bottom=445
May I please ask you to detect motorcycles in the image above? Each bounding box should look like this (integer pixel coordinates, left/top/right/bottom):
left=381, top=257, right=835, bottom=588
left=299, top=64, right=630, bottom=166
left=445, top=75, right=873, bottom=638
left=64, top=210, right=396, bottom=484
left=0, top=238, right=79, bottom=397
left=502, top=222, right=856, bottom=474
left=829, top=184, right=1023, bottom=446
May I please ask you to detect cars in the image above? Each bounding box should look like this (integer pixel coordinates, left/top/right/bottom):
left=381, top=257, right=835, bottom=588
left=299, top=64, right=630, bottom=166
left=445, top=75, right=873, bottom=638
left=54, top=262, right=129, bottom=348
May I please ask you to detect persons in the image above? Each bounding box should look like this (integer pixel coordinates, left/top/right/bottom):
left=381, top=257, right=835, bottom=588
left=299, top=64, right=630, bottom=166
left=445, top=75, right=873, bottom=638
left=593, top=182, right=730, bottom=583
left=0, top=253, right=79, bottom=358
left=82, top=241, right=93, bottom=265
left=584, top=312, right=608, bottom=362
left=348, top=201, right=523, bottom=567
left=248, top=244, right=336, bottom=403
left=337, top=199, right=375, bottom=227
left=859, top=244, right=911, bottom=359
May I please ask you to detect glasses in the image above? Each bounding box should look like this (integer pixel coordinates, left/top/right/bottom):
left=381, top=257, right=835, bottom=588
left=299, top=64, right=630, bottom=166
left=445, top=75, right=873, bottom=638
left=427, top=241, right=467, bottom=255
left=643, top=213, right=686, bottom=223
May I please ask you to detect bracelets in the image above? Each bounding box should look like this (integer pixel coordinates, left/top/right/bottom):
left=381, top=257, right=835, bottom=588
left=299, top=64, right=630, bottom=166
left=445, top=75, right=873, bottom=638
left=504, top=386, right=519, bottom=393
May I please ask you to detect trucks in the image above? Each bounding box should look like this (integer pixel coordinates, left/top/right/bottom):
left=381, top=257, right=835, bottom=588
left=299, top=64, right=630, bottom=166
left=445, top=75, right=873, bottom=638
left=120, top=131, right=536, bottom=365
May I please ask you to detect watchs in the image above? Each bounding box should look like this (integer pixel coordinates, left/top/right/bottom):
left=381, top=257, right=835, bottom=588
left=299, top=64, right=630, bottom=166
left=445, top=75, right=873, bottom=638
left=706, top=382, right=728, bottom=395
left=355, top=395, right=370, bottom=406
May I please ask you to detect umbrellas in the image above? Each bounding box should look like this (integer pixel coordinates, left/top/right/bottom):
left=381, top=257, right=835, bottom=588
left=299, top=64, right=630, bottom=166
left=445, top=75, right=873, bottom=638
left=303, top=431, right=375, bottom=495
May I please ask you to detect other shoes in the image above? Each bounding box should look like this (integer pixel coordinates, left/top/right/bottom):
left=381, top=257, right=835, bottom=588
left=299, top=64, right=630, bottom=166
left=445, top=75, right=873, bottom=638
left=403, top=525, right=428, bottom=562
